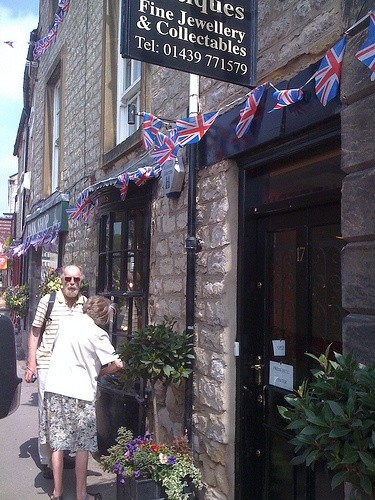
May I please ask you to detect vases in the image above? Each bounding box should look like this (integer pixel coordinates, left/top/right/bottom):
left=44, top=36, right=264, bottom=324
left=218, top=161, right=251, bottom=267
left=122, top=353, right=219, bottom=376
left=117, top=470, right=196, bottom=500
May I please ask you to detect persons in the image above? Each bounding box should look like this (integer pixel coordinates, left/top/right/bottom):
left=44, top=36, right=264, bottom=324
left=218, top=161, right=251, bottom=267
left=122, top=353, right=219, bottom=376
left=24, top=265, right=90, bottom=480
left=38, top=295, right=124, bottom=500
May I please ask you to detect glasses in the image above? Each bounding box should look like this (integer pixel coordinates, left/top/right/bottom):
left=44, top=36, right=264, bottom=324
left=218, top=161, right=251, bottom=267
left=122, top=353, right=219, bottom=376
left=65, top=277, right=81, bottom=282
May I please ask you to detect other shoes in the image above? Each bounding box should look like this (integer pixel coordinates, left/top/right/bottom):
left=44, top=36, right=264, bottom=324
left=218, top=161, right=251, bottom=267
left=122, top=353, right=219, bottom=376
left=63, top=457, right=76, bottom=469
left=43, top=465, right=54, bottom=479
left=51, top=493, right=63, bottom=500
left=82, top=492, right=103, bottom=500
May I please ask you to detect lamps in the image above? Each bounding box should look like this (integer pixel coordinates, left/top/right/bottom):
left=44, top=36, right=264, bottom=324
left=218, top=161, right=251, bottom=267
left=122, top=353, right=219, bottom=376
left=128, top=103, right=146, bottom=125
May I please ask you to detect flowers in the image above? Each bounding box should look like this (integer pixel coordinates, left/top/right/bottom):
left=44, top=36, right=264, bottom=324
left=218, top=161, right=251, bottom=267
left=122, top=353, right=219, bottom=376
left=100, top=424, right=208, bottom=500
left=38, top=267, right=89, bottom=299
left=3, top=283, right=30, bottom=319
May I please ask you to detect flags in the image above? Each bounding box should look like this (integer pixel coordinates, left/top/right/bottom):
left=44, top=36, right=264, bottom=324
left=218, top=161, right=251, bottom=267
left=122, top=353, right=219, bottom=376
left=141, top=110, right=219, bottom=166
left=13, top=223, right=60, bottom=256
left=355, top=7, right=375, bottom=82
left=267, top=85, right=305, bottom=115
left=116, top=164, right=163, bottom=201
left=65, top=191, right=93, bottom=226
left=236, top=80, right=269, bottom=140
left=3, top=0, right=70, bottom=61
left=315, top=36, right=345, bottom=108
